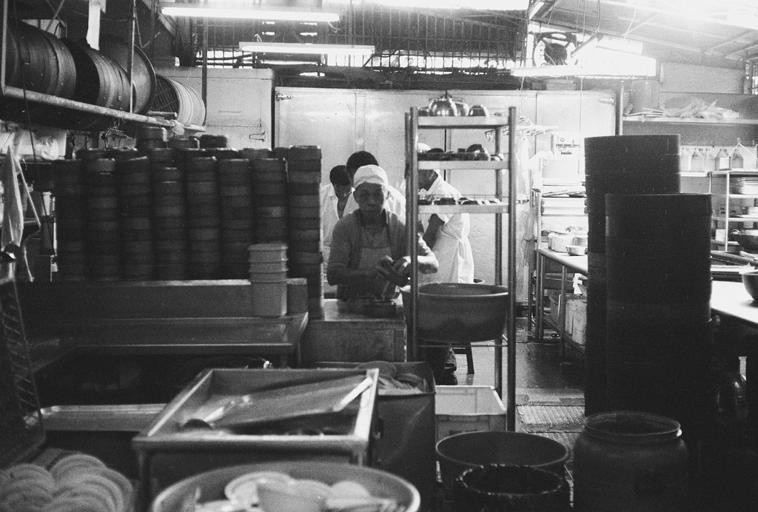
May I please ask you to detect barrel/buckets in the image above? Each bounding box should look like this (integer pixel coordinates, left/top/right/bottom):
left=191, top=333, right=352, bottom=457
left=247, top=243, right=289, bottom=317
left=436, top=430, right=571, bottom=489
left=573, top=409, right=689, bottom=512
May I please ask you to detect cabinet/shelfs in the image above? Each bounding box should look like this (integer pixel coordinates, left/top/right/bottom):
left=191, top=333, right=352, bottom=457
left=618, top=85, right=758, bottom=254
left=404, top=105, right=517, bottom=435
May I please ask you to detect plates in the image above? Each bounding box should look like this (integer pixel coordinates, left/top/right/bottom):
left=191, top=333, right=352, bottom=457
left=355, top=359, right=430, bottom=396
left=2, top=453, right=133, bottom=512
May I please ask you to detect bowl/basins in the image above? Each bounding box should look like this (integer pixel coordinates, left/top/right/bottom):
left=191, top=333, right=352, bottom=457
left=176, top=471, right=395, bottom=512
left=741, top=269, right=758, bottom=305
left=467, top=144, right=491, bottom=160
left=418, top=195, right=500, bottom=204
left=468, top=104, right=488, bottom=116
left=400, top=278, right=508, bottom=338
left=732, top=229, right=758, bottom=252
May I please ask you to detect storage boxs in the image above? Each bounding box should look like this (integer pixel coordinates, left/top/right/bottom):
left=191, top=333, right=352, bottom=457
left=432, top=384, right=508, bottom=443
left=131, top=359, right=380, bottom=497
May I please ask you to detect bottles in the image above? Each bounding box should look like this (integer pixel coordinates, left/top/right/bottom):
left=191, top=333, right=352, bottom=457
left=547, top=229, right=569, bottom=253
left=680, top=142, right=748, bottom=174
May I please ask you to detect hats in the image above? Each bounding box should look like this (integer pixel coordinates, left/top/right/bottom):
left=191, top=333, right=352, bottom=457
left=417, top=142, right=430, bottom=152
left=353, top=165, right=388, bottom=190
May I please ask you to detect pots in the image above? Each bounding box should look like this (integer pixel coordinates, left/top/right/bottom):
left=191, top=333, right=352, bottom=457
left=567, top=246, right=588, bottom=256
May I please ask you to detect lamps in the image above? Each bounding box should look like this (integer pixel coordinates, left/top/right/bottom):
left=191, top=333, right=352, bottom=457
left=160, top=0, right=378, bottom=59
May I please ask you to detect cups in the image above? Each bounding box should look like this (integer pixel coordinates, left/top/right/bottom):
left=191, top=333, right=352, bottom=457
left=573, top=235, right=588, bottom=246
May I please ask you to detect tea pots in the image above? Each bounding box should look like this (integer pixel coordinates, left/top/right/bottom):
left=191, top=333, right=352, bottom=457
left=432, top=99, right=457, bottom=116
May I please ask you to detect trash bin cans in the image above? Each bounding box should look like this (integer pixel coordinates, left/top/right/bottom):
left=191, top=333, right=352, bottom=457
left=454, top=464, right=566, bottom=512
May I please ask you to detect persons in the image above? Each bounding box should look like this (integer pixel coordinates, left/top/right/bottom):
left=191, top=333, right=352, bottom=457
left=319, top=166, right=354, bottom=270
left=343, top=150, right=407, bottom=224
left=326, top=165, right=440, bottom=302
left=400, top=140, right=431, bottom=197
left=419, top=169, right=475, bottom=384
